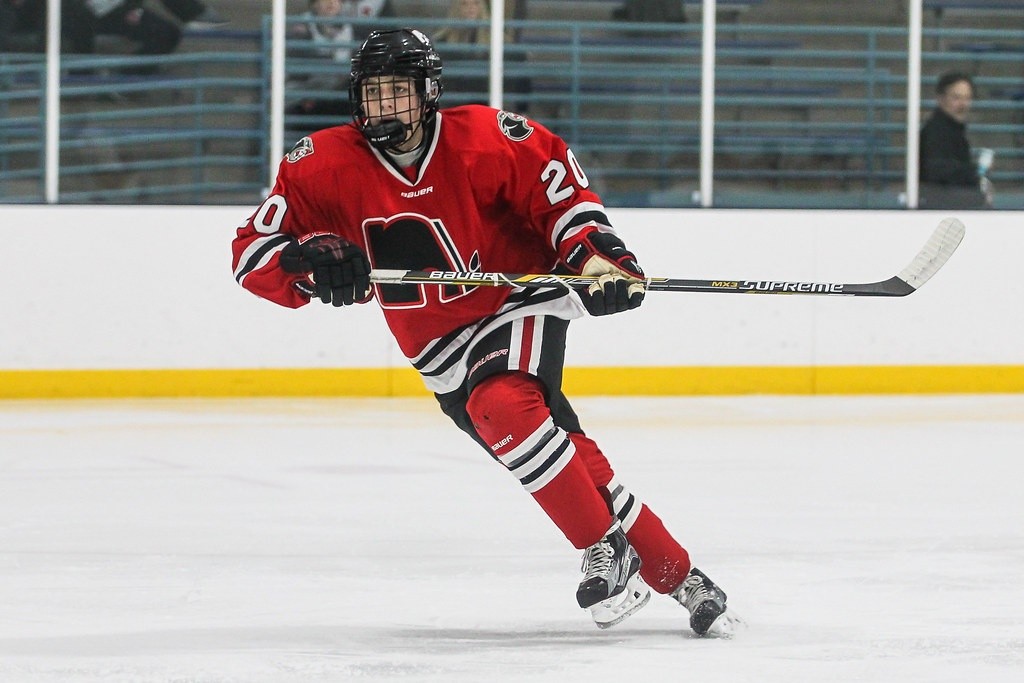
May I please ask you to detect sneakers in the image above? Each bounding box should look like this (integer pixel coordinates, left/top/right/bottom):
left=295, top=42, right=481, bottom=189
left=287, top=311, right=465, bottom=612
left=667, top=566, right=748, bottom=641
left=575, top=513, right=651, bottom=630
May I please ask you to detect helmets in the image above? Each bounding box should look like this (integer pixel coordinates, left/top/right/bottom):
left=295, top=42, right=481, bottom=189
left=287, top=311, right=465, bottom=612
left=348, top=27, right=444, bottom=151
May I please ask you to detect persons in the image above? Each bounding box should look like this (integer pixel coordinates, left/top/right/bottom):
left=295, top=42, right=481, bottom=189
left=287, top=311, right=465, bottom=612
left=285, top=0, right=353, bottom=138
left=232, top=28, right=743, bottom=639
left=918, top=70, right=992, bottom=211
left=0, top=0, right=205, bottom=106
left=430, top=0, right=537, bottom=113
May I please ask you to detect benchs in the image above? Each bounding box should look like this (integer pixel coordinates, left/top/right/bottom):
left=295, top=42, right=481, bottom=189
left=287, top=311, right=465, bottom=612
left=0, top=0, right=1024, bottom=208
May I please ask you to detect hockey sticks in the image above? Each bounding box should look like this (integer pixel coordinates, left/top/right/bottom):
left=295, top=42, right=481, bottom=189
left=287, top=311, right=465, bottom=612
left=304, top=215, right=967, bottom=298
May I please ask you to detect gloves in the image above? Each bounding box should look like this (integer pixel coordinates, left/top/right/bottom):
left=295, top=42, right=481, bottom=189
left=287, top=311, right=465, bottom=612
left=279, top=230, right=370, bottom=308
left=572, top=233, right=645, bottom=317
